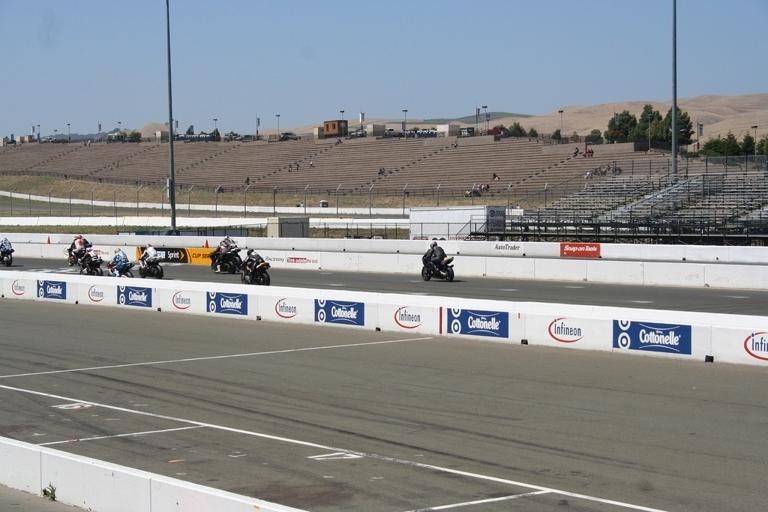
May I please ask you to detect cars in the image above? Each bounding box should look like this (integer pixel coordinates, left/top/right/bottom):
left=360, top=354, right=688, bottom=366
left=235, top=136, right=243, bottom=141
left=279, top=132, right=301, bottom=140
left=351, top=128, right=437, bottom=137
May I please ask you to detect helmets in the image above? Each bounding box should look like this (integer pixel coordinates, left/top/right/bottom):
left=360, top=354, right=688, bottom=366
left=74, top=234, right=152, bottom=253
left=430, top=241, right=437, bottom=248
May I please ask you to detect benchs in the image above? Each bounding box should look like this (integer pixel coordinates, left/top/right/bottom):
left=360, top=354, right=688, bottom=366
left=0, top=145, right=768, bottom=245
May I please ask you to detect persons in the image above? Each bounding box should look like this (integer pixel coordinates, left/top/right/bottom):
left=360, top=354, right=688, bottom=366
left=138, top=243, right=158, bottom=269
left=214, top=234, right=237, bottom=273
left=465, top=184, right=489, bottom=196
left=424, top=242, right=447, bottom=271
left=108, top=248, right=128, bottom=277
left=69, top=235, right=98, bottom=273
left=239, top=249, right=265, bottom=275
left=0, top=238, right=12, bottom=256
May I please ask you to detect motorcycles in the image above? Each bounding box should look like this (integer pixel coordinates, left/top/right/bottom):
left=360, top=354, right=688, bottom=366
left=0, top=248, right=16, bottom=268
left=420, top=248, right=456, bottom=282
left=208, top=244, right=272, bottom=286
left=65, top=241, right=164, bottom=280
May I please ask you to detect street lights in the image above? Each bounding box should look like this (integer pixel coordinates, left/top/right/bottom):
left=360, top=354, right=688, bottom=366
left=750, top=125, right=759, bottom=163
left=558, top=110, right=566, bottom=138
left=275, top=114, right=281, bottom=142
left=339, top=111, right=345, bottom=139
left=32, top=121, right=124, bottom=144
left=213, top=119, right=218, bottom=142
left=481, top=105, right=490, bottom=135
left=401, top=109, right=408, bottom=140
